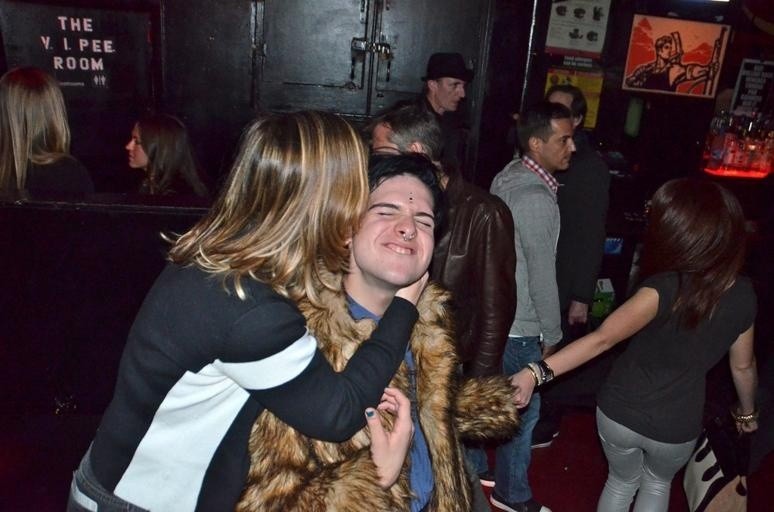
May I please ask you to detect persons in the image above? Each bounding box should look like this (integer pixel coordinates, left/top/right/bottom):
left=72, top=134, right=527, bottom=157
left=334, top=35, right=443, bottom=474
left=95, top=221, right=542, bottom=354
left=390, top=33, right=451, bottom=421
left=626, top=34, right=719, bottom=92
left=360, top=51, right=473, bottom=141
left=125, top=112, right=208, bottom=200
left=508, top=176, right=760, bottom=512
left=235, top=152, right=510, bottom=512
left=365, top=101, right=517, bottom=512
left=530, top=85, right=609, bottom=449
left=461, top=101, right=575, bottom=512
left=0, top=68, right=94, bottom=193
left=65, top=110, right=429, bottom=512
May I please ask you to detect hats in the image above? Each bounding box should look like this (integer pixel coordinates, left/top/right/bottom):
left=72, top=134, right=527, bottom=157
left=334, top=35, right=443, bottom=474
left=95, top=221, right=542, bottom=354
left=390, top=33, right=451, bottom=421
left=420, top=50, right=475, bottom=84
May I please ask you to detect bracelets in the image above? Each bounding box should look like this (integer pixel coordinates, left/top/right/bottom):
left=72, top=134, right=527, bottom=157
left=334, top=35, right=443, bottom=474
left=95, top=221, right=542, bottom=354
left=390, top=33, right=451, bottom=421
left=527, top=365, right=538, bottom=384
left=729, top=409, right=760, bottom=422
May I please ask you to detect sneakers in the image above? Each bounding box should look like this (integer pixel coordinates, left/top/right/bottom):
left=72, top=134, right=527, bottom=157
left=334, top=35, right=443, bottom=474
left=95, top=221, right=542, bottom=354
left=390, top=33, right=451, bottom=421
left=530, top=433, right=554, bottom=450
left=553, top=425, right=560, bottom=437
left=476, top=467, right=496, bottom=488
left=489, top=489, right=552, bottom=512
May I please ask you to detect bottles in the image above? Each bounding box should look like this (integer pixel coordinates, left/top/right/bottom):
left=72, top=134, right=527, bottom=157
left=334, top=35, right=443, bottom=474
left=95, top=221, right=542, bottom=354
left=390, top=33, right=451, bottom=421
left=709, top=107, right=774, bottom=174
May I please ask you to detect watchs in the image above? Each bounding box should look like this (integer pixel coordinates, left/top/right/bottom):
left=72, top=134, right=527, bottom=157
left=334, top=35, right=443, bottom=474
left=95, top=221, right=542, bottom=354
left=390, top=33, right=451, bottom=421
left=534, top=360, right=555, bottom=382
